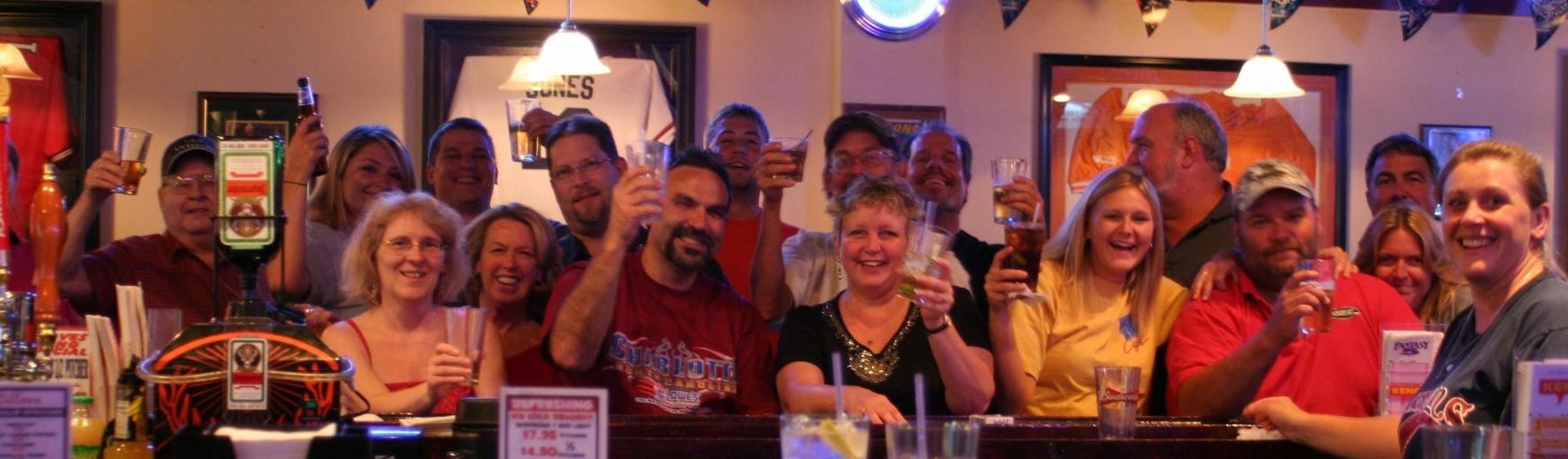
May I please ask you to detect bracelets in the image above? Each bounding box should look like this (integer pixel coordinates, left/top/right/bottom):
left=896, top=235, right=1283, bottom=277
left=826, top=388, right=837, bottom=414
left=282, top=180, right=308, bottom=187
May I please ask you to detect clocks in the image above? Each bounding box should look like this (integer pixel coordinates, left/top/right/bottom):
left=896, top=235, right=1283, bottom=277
left=840, top=0, right=948, bottom=40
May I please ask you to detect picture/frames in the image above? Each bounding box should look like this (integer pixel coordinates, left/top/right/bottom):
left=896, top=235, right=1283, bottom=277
left=843, top=100, right=947, bottom=132
left=422, top=17, right=696, bottom=203
left=196, top=91, right=317, bottom=141
left=1038, top=52, right=1353, bottom=260
left=1, top=0, right=103, bottom=307
left=1420, top=124, right=1492, bottom=169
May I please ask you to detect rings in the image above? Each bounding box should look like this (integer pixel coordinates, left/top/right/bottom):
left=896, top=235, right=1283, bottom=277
left=861, top=407, right=869, bottom=415
left=1201, top=271, right=1209, bottom=275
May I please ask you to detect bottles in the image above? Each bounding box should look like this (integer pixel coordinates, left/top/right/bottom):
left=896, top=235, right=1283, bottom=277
left=213, top=134, right=284, bottom=296
left=28, top=162, right=64, bottom=321
left=113, top=368, right=136, bottom=442
left=69, top=395, right=105, bottom=459
left=296, top=76, right=328, bottom=176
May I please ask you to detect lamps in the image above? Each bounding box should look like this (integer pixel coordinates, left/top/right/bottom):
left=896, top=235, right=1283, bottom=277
left=1222, top=0, right=1307, bottom=99
left=497, top=56, right=568, bottom=92
left=1113, top=89, right=1170, bottom=122
left=532, top=0, right=611, bottom=76
left=0, top=43, right=43, bottom=81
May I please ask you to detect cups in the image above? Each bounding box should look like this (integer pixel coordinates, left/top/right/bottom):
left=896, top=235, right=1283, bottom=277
left=907, top=198, right=940, bottom=253
left=895, top=220, right=956, bottom=305
left=776, top=412, right=870, bottom=459
left=1003, top=216, right=1046, bottom=299
left=625, top=140, right=670, bottom=220
left=443, top=306, right=488, bottom=385
left=505, top=98, right=541, bottom=162
left=1293, top=257, right=1336, bottom=333
left=1094, top=365, right=1141, bottom=440
left=147, top=308, right=182, bottom=358
left=109, top=126, right=153, bottom=195
left=768, top=137, right=808, bottom=182
left=989, top=157, right=1027, bottom=223
left=883, top=415, right=980, bottom=459
left=1420, top=424, right=1525, bottom=459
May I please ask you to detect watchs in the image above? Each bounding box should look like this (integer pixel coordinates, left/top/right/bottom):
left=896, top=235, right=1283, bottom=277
left=923, top=314, right=952, bottom=335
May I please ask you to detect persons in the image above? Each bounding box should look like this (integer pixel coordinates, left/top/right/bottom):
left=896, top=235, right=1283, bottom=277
left=1363, top=132, right=1442, bottom=218
left=773, top=174, right=996, bottom=426
left=318, top=189, right=509, bottom=417
left=1189, top=198, right=1473, bottom=334
left=537, top=144, right=781, bottom=416
left=7, top=141, right=22, bottom=247
left=1126, top=100, right=1243, bottom=418
left=265, top=114, right=415, bottom=322
left=32, top=134, right=276, bottom=360
left=443, top=202, right=578, bottom=388
left=984, top=166, right=1360, bottom=419
left=425, top=103, right=1046, bottom=311
left=1165, top=160, right=1427, bottom=420
left=1242, top=138, right=1568, bottom=457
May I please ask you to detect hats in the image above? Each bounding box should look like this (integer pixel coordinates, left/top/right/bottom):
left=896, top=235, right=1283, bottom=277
left=825, top=112, right=902, bottom=161
left=1235, top=159, right=1318, bottom=212
left=161, top=135, right=216, bottom=176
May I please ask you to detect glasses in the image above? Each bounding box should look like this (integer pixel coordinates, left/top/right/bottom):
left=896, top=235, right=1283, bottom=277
left=552, top=156, right=612, bottom=183
left=829, top=149, right=890, bottom=172
left=170, top=174, right=216, bottom=196
left=689, top=388, right=752, bottom=418
left=383, top=236, right=449, bottom=257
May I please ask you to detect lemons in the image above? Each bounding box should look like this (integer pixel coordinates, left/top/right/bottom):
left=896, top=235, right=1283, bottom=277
left=821, top=418, right=868, bottom=459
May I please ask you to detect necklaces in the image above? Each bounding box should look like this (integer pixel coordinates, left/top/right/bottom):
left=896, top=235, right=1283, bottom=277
left=866, top=339, right=874, bottom=345
